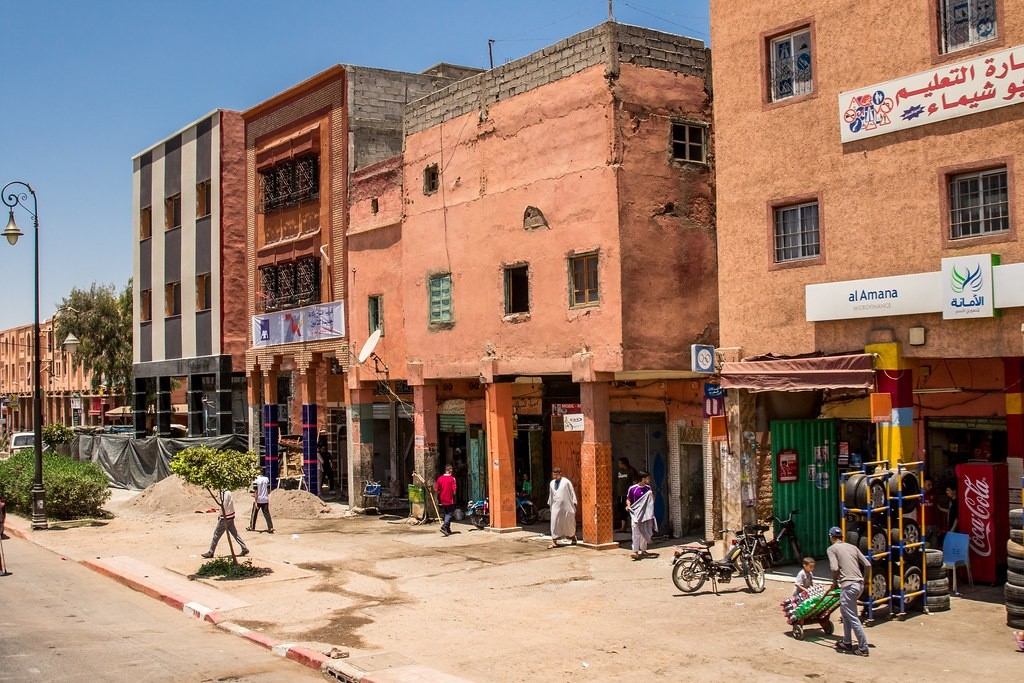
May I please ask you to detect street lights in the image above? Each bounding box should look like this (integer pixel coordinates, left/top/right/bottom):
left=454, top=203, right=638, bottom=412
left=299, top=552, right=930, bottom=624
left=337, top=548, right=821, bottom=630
left=0, top=180, right=49, bottom=531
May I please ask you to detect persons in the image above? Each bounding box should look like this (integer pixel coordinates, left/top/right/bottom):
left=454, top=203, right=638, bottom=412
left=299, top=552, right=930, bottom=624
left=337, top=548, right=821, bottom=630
left=793, top=558, right=815, bottom=595
left=246, top=466, right=274, bottom=532
left=523, top=474, right=540, bottom=521
left=916, top=478, right=958, bottom=548
left=0, top=495, right=6, bottom=535
left=614, top=457, right=638, bottom=532
left=436, top=464, right=457, bottom=536
left=201, top=491, right=249, bottom=558
left=827, top=528, right=871, bottom=656
left=548, top=468, right=578, bottom=549
left=626, top=471, right=654, bottom=559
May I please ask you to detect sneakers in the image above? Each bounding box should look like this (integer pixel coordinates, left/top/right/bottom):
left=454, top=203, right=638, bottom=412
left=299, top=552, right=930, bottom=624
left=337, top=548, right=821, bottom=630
left=853, top=648, right=869, bottom=657
left=835, top=641, right=853, bottom=650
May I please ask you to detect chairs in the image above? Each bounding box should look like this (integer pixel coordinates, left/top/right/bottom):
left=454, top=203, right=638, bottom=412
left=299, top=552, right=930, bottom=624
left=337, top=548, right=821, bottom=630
left=938, top=531, right=973, bottom=593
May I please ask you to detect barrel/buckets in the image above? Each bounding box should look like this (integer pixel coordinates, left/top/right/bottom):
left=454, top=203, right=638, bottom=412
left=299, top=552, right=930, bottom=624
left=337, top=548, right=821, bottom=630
left=367, top=485, right=381, bottom=495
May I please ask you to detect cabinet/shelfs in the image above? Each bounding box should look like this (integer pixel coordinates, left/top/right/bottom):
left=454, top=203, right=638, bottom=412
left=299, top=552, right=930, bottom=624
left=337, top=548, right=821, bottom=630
left=840, top=459, right=930, bottom=626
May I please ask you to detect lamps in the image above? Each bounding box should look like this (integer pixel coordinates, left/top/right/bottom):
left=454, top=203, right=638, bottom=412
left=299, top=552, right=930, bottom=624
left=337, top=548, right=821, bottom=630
left=913, top=387, right=963, bottom=394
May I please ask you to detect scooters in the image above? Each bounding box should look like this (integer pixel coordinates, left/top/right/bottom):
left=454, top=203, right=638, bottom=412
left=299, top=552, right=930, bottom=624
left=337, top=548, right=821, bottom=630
left=736, top=509, right=804, bottom=577
left=466, top=488, right=539, bottom=529
left=671, top=522, right=766, bottom=594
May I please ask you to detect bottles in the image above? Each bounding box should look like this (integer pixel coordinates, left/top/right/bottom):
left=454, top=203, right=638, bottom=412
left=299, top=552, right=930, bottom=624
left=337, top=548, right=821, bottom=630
left=779, top=583, right=842, bottom=625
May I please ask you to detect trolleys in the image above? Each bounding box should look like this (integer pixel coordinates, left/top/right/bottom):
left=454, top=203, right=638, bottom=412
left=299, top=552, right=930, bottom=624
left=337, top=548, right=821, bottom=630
left=791, top=581, right=841, bottom=641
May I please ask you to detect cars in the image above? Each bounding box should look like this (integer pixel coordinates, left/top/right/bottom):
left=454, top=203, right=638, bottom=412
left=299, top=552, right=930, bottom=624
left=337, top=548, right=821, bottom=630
left=5, top=432, right=34, bottom=458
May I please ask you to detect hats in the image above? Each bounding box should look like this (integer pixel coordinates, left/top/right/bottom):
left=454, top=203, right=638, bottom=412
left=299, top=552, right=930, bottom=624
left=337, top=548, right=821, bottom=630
left=829, top=527, right=843, bottom=536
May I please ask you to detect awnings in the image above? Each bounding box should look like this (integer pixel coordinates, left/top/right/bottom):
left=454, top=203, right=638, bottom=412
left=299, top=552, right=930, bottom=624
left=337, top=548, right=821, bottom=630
left=720, top=354, right=874, bottom=389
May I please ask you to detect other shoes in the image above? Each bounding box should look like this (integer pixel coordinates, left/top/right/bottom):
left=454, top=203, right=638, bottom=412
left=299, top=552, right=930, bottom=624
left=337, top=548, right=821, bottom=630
left=267, top=528, right=274, bottom=533
left=201, top=552, right=214, bottom=558
left=572, top=536, right=577, bottom=545
left=246, top=527, right=256, bottom=531
left=239, top=548, right=249, bottom=556
left=547, top=544, right=559, bottom=549
left=440, top=525, right=450, bottom=537
left=631, top=553, right=639, bottom=559
left=642, top=551, right=649, bottom=556
